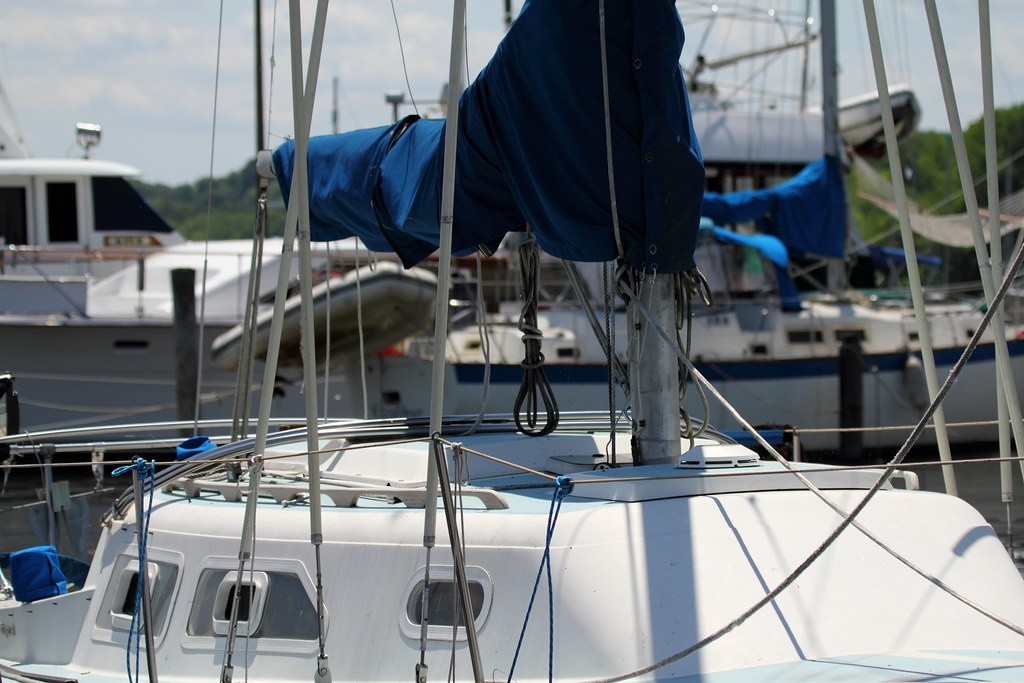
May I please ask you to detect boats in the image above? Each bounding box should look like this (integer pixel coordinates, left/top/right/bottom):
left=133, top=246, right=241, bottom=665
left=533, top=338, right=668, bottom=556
left=0, top=125, right=460, bottom=443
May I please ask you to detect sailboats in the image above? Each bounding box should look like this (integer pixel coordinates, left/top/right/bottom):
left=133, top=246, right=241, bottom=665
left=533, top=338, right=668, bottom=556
left=0, top=0, right=1024, bottom=683
left=357, top=0, right=1024, bottom=455
